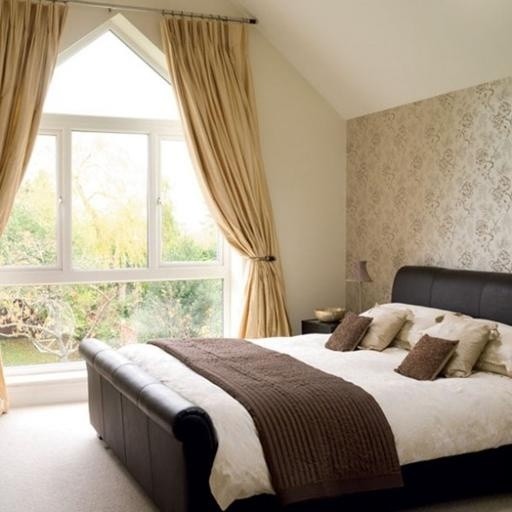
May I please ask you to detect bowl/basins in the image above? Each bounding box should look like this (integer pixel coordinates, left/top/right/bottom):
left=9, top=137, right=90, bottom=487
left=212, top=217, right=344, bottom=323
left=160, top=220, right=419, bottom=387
left=313, top=306, right=345, bottom=323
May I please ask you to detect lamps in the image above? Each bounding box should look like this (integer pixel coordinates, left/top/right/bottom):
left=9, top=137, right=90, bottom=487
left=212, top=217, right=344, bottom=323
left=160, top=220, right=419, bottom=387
left=344, top=261, right=374, bottom=313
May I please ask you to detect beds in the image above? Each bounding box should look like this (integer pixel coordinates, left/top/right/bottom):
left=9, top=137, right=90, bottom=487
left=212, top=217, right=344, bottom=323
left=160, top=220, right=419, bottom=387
left=77, top=266, right=512, bottom=512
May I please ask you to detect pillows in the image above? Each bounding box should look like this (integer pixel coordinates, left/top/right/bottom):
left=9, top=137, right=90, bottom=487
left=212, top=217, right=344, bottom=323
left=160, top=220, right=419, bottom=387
left=393, top=333, right=460, bottom=381
left=324, top=309, right=373, bottom=352
left=356, top=302, right=410, bottom=352
left=457, top=314, right=512, bottom=379
left=381, top=301, right=462, bottom=351
left=416, top=312, right=498, bottom=377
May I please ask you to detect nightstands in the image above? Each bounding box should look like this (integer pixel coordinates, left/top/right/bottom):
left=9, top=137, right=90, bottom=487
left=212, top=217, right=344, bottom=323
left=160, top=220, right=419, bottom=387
left=301, top=318, right=341, bottom=333
